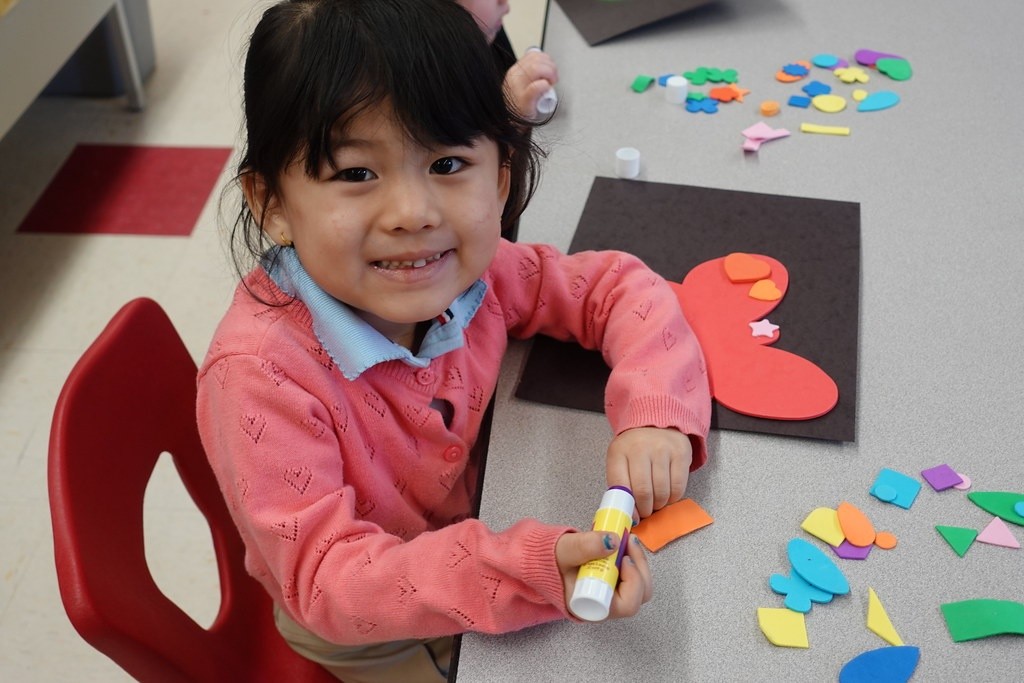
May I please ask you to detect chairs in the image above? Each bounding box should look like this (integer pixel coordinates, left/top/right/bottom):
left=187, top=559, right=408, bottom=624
left=45, top=297, right=338, bottom=683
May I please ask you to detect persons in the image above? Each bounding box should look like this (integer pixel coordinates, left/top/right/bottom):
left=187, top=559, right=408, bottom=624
left=196, top=0, right=711, bottom=683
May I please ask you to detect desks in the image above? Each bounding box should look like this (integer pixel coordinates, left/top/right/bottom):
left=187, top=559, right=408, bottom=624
left=457, top=0, right=1024, bottom=683
left=0, top=0, right=147, bottom=141
